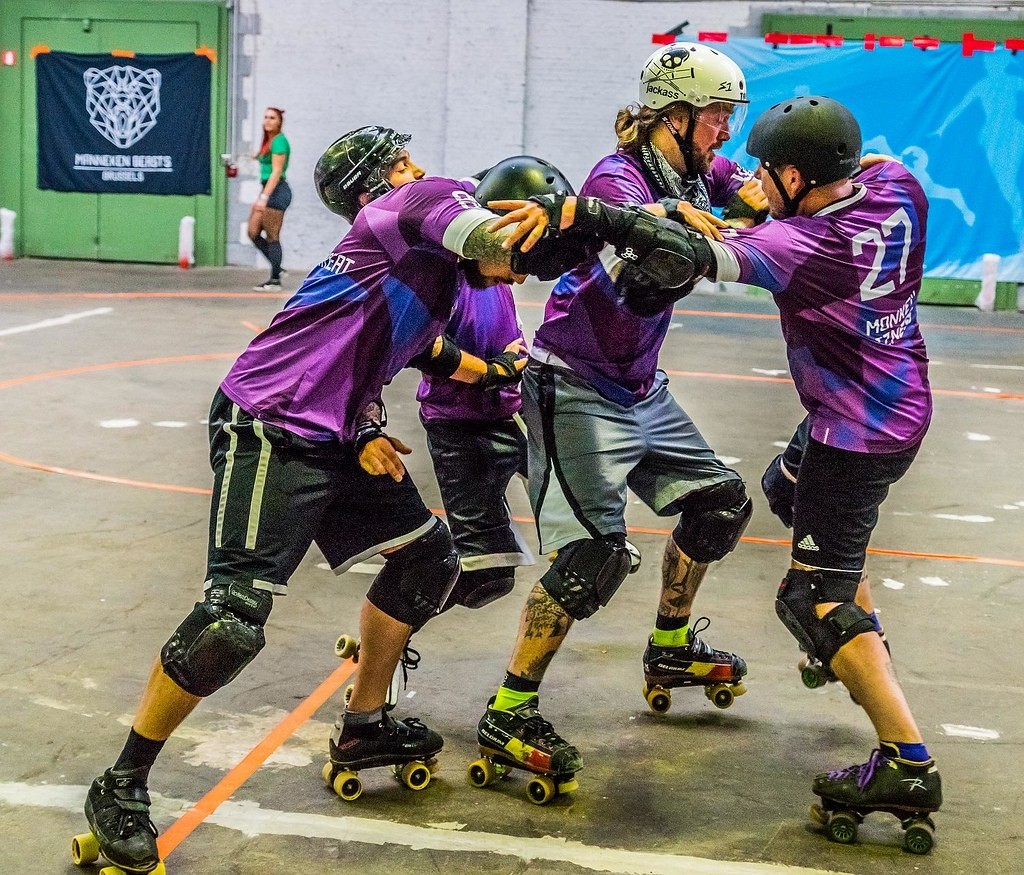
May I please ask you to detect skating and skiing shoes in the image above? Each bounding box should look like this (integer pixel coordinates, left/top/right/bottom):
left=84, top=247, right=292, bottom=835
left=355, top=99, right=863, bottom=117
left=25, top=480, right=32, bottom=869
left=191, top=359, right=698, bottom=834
left=642, top=617, right=748, bottom=714
left=72, top=766, right=165, bottom=875
left=335, top=634, right=422, bottom=712
left=321, top=712, right=444, bottom=801
left=798, top=627, right=891, bottom=704
left=467, top=695, right=584, bottom=804
left=811, top=740, right=943, bottom=854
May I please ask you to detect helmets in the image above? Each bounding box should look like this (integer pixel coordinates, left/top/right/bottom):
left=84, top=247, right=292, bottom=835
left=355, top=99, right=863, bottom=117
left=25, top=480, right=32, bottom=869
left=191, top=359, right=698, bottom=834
left=639, top=42, right=750, bottom=110
left=314, top=125, right=412, bottom=224
left=746, top=96, right=862, bottom=188
left=474, top=156, right=578, bottom=225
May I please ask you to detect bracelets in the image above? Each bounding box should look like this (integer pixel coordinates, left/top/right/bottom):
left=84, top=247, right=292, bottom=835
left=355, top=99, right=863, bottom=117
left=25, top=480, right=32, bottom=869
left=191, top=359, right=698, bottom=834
left=260, top=193, right=269, bottom=199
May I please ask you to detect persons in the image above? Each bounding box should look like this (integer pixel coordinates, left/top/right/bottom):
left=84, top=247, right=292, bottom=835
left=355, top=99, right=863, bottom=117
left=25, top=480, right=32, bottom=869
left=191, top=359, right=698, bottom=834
left=311, top=124, right=530, bottom=630
left=476, top=43, right=769, bottom=772
left=247, top=108, right=292, bottom=292
left=490, top=94, right=943, bottom=813
left=83, top=154, right=576, bottom=871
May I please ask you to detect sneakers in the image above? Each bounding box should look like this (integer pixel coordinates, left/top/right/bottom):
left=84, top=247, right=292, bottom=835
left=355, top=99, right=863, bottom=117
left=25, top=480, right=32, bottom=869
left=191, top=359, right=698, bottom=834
left=278, top=268, right=289, bottom=282
left=252, top=281, right=282, bottom=293
